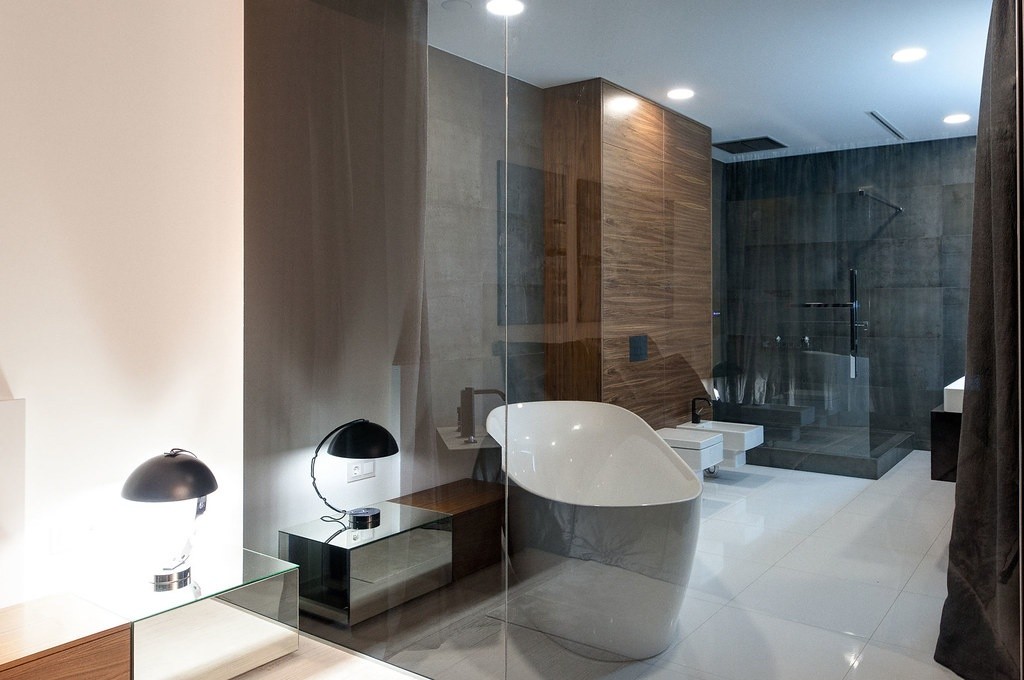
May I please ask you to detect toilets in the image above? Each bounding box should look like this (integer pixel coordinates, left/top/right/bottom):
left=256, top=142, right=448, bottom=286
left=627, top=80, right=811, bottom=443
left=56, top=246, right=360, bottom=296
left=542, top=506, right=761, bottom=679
left=654, top=427, right=722, bottom=484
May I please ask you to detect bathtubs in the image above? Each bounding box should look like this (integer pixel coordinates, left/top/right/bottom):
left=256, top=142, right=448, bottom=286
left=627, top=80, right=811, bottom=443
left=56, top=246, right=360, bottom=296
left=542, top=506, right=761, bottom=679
left=486, top=400, right=704, bottom=662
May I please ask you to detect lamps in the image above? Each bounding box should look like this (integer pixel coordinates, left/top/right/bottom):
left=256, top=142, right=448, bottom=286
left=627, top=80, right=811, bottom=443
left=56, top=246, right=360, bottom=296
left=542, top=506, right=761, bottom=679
left=309, top=418, right=399, bottom=527
left=118, top=448, right=220, bottom=584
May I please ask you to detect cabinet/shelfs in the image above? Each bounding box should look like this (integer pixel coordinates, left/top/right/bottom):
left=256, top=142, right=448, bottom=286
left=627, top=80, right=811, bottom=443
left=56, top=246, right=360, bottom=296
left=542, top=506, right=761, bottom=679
left=279, top=477, right=521, bottom=628
left=0, top=542, right=299, bottom=680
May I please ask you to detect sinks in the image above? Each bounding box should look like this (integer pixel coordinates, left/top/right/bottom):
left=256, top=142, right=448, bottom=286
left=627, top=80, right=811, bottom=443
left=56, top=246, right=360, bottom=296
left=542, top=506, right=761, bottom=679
left=675, top=418, right=763, bottom=468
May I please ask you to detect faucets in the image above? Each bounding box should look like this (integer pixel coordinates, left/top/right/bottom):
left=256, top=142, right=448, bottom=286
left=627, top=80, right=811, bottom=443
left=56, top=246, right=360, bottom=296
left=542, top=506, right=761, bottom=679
left=459, top=387, right=505, bottom=436
left=691, top=397, right=714, bottom=423
